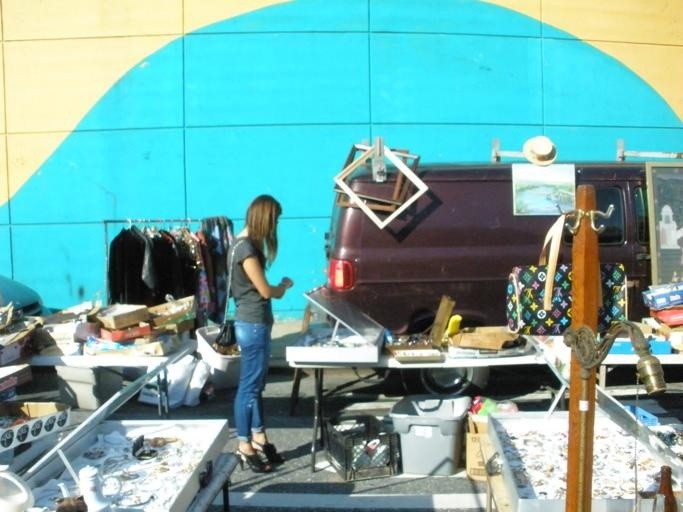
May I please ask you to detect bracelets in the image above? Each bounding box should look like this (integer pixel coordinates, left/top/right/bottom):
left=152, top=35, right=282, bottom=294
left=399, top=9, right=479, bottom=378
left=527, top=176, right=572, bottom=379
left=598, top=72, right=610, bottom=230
left=279, top=281, right=289, bottom=287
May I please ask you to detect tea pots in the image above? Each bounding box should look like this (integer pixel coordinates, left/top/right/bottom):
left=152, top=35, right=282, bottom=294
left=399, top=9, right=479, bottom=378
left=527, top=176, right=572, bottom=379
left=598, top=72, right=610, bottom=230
left=56, top=464, right=123, bottom=511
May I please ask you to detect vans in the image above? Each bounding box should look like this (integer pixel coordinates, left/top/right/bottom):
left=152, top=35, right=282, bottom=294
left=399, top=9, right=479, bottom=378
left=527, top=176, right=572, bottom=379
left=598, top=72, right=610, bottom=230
left=317, top=162, right=681, bottom=399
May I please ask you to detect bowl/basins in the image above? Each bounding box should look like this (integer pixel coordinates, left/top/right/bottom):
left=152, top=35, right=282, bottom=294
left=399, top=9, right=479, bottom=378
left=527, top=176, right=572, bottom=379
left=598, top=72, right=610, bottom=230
left=420, top=368, right=474, bottom=398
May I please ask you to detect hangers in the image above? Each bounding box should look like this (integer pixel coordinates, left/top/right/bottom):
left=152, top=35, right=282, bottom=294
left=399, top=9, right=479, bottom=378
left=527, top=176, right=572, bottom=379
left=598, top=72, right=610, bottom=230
left=124, top=218, right=192, bottom=231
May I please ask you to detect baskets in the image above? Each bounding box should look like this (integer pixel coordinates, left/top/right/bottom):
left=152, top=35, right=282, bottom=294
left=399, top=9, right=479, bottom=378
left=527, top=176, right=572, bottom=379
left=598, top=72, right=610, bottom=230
left=320, top=414, right=394, bottom=482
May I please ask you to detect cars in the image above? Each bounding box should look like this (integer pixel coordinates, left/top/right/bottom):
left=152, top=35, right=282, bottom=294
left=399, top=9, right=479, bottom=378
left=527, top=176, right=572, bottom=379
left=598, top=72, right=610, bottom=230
left=1, top=272, right=51, bottom=321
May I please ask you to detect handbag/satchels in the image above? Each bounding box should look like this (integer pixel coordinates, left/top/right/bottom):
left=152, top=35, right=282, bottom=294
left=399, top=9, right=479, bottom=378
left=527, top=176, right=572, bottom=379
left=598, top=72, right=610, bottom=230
left=507, top=261, right=629, bottom=337
left=138, top=354, right=211, bottom=408
left=215, top=321, right=235, bottom=346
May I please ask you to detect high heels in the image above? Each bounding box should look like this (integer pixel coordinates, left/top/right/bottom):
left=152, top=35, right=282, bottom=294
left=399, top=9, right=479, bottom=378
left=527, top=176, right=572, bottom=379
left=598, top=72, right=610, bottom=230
left=234, top=448, right=272, bottom=473
left=252, top=438, right=285, bottom=466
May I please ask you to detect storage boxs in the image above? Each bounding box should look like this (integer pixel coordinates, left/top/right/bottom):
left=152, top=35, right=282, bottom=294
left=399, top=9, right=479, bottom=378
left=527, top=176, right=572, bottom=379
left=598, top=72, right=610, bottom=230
left=145, top=294, right=199, bottom=326
left=92, top=303, right=150, bottom=330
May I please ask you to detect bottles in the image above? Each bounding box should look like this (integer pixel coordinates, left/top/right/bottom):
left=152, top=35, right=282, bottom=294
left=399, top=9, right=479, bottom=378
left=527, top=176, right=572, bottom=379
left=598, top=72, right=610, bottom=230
left=652, top=465, right=678, bottom=512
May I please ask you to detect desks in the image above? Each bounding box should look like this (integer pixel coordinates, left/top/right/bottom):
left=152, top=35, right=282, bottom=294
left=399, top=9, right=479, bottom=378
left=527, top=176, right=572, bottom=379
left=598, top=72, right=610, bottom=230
left=290, top=320, right=683, bottom=477
left=1, top=325, right=241, bottom=511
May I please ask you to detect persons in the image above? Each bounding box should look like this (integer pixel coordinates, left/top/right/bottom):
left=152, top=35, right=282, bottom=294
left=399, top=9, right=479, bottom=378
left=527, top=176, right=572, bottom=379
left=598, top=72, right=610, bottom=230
left=225, top=194, right=295, bottom=473
